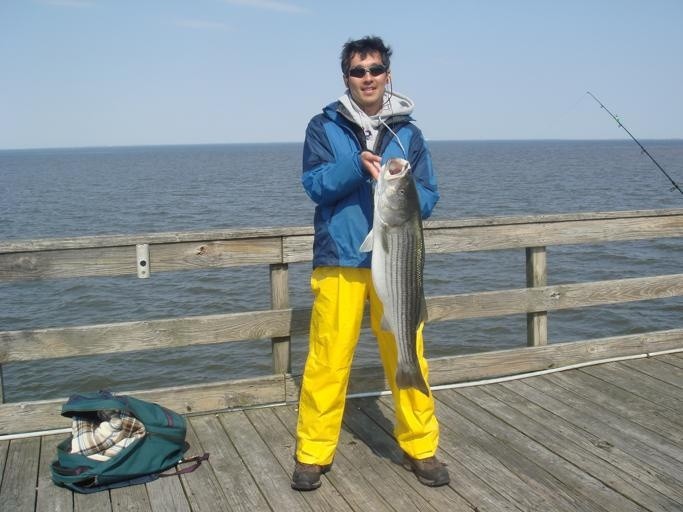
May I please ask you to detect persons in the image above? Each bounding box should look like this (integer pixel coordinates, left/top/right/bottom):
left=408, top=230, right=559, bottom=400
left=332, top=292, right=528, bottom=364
left=288, top=34, right=449, bottom=492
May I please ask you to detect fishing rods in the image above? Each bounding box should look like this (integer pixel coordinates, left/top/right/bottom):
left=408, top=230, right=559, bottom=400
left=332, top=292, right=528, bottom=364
left=585, top=90, right=683, bottom=199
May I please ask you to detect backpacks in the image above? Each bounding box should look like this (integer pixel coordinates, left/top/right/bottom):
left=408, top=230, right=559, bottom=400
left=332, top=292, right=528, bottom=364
left=51, top=389, right=187, bottom=494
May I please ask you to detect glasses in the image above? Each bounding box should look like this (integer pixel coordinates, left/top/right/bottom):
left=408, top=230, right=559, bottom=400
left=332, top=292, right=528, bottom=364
left=348, top=63, right=387, bottom=78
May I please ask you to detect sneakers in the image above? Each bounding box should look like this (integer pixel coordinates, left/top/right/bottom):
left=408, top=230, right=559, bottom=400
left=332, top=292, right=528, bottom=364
left=404, top=452, right=451, bottom=487
left=291, top=459, right=333, bottom=491
